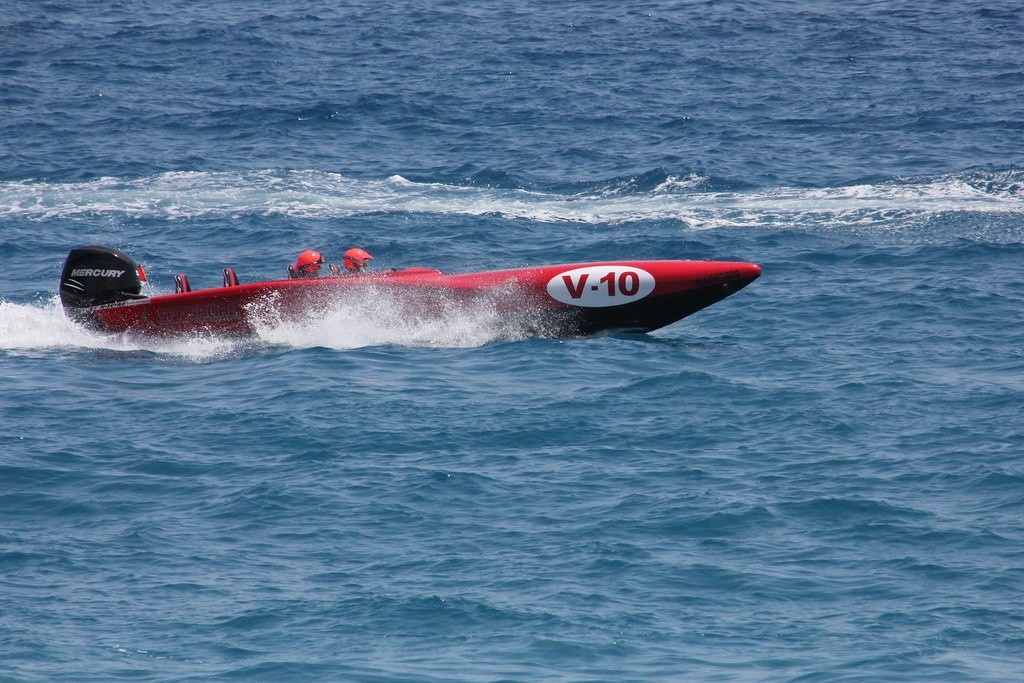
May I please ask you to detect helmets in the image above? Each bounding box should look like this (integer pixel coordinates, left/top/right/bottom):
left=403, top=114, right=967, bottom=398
left=296, top=249, right=324, bottom=274
left=343, top=248, right=374, bottom=270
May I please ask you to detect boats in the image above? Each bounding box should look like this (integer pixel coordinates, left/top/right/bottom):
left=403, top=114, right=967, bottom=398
left=61, top=241, right=762, bottom=343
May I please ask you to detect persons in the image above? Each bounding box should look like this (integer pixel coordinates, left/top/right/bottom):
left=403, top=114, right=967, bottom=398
left=341, top=248, right=376, bottom=280
left=294, top=250, right=327, bottom=282
